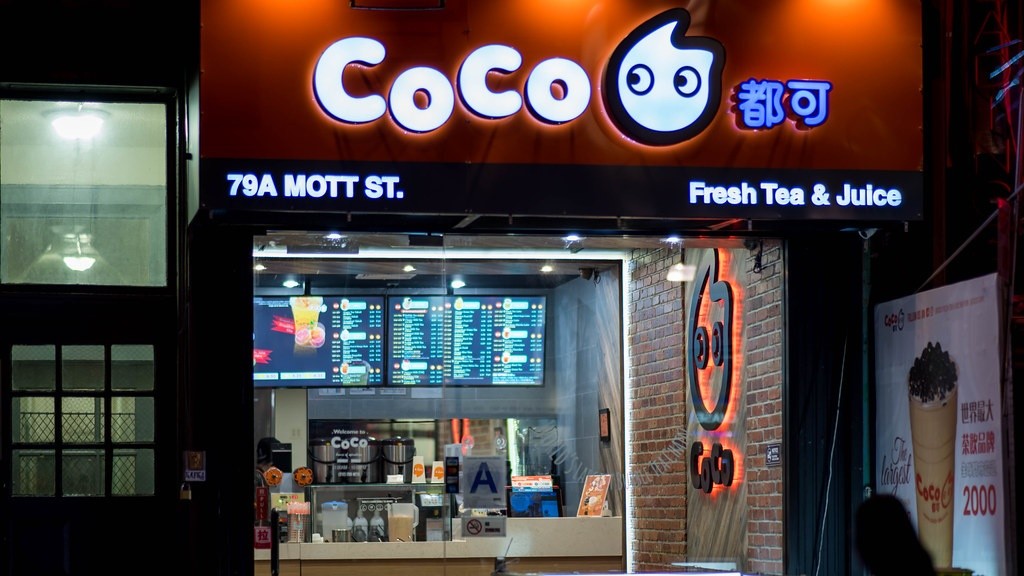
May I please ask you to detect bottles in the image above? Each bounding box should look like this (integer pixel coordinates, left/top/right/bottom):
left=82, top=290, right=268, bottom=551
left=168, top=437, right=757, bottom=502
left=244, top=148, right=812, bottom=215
left=354, top=506, right=368, bottom=540
left=370, top=509, right=384, bottom=541
left=276, top=496, right=288, bottom=543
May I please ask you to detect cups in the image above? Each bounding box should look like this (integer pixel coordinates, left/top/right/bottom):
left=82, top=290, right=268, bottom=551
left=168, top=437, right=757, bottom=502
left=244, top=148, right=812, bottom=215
left=431, top=461, right=445, bottom=484
left=332, top=529, right=350, bottom=542
left=291, top=305, right=321, bottom=330
left=411, top=456, right=427, bottom=484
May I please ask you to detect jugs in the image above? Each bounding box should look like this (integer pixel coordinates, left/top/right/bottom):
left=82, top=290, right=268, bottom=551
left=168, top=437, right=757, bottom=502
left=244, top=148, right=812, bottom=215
left=321, top=501, right=348, bottom=542
left=388, top=503, right=420, bottom=542
